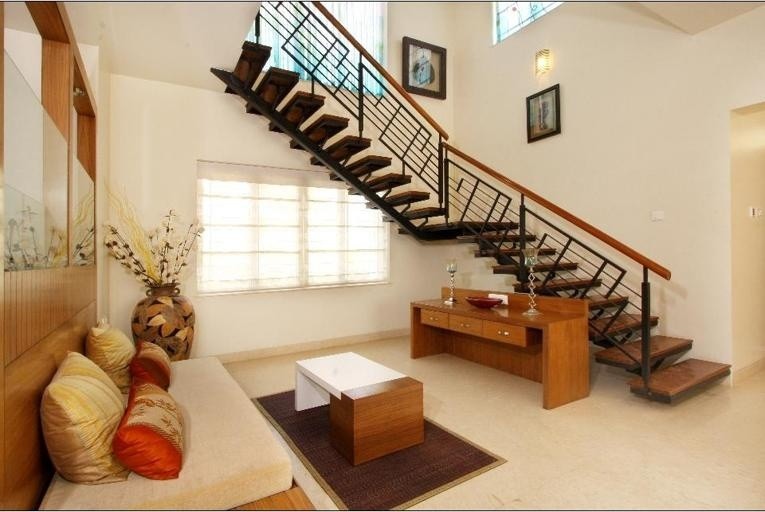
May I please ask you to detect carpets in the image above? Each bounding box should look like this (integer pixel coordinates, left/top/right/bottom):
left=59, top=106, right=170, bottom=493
left=252, top=388, right=508, bottom=511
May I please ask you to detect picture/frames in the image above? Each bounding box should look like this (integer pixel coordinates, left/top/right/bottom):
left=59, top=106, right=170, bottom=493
left=526, top=84, right=562, bottom=144
left=402, top=36, right=447, bottom=99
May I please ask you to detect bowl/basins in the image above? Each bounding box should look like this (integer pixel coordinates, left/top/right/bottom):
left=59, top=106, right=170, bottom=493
left=464, top=296, right=503, bottom=309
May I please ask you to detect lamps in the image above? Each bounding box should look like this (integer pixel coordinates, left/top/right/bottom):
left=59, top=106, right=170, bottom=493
left=535, top=48, right=551, bottom=76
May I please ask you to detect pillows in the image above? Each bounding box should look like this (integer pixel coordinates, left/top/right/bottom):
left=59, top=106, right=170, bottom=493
left=40, top=316, right=184, bottom=485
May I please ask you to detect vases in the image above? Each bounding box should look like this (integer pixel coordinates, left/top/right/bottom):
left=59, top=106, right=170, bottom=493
left=132, top=282, right=195, bottom=361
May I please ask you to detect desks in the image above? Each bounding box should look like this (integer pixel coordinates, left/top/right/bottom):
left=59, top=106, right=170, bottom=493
left=409, top=288, right=590, bottom=410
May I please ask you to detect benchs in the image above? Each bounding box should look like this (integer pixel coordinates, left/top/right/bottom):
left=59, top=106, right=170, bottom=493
left=37, top=355, right=316, bottom=511
